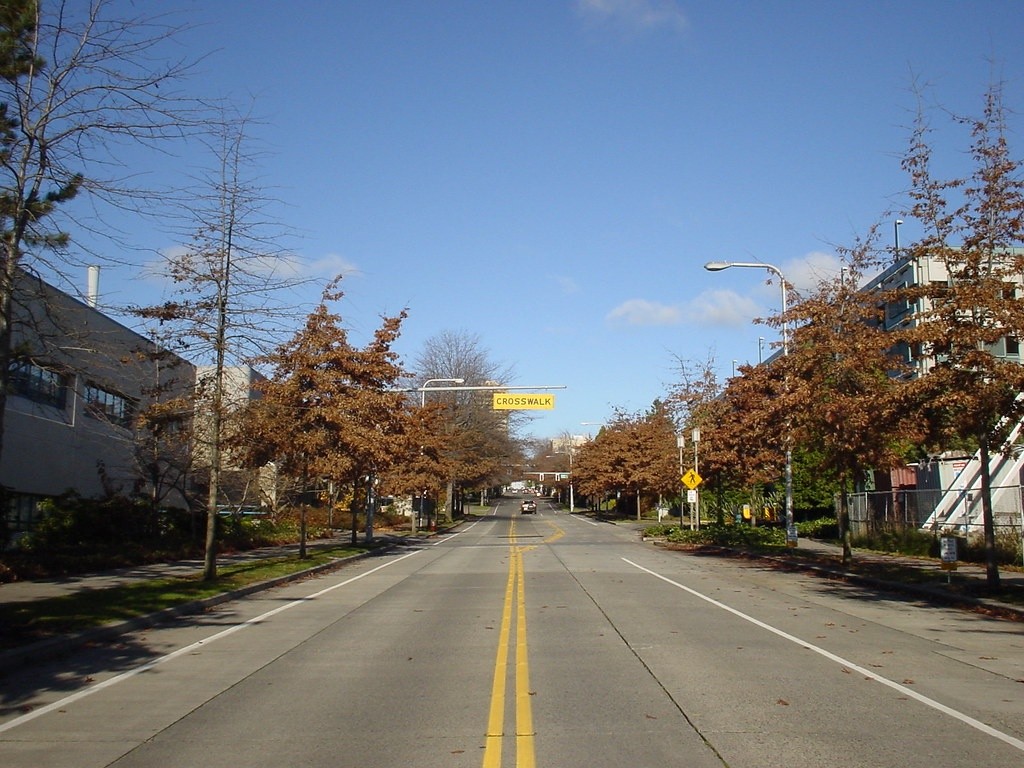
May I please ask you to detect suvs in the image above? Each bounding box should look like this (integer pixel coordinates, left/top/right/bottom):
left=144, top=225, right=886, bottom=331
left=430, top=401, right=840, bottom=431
left=521, top=500, right=537, bottom=514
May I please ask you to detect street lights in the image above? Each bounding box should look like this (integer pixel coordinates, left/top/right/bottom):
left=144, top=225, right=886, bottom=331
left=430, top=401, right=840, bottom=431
left=732, top=360, right=737, bottom=378
left=758, top=336, right=765, bottom=363
left=894, top=219, right=903, bottom=264
left=555, top=451, right=574, bottom=512
left=840, top=266, right=849, bottom=287
left=703, top=259, right=795, bottom=552
left=419, top=377, right=465, bottom=528
left=579, top=423, right=640, bottom=521
